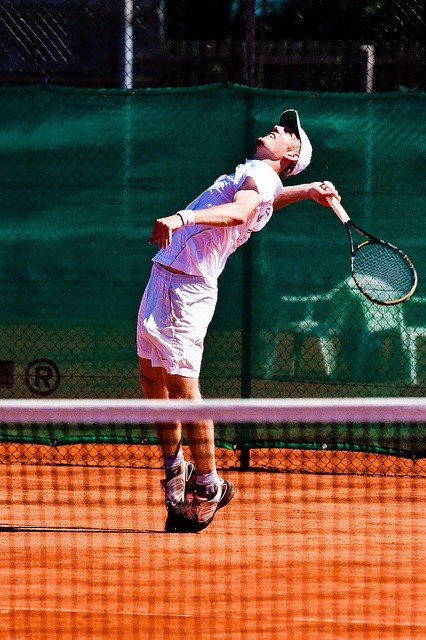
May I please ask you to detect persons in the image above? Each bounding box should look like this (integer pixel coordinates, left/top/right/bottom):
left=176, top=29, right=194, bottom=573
left=135, top=109, right=341, bottom=531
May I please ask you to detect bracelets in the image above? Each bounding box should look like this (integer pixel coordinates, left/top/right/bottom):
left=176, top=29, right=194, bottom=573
left=177, top=209, right=195, bottom=226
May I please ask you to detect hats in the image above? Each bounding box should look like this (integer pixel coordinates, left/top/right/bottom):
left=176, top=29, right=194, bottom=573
left=279, top=109, right=313, bottom=177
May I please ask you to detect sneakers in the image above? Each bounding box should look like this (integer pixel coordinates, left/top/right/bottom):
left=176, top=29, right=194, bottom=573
left=178, top=479, right=233, bottom=533
left=159, top=462, right=197, bottom=532
